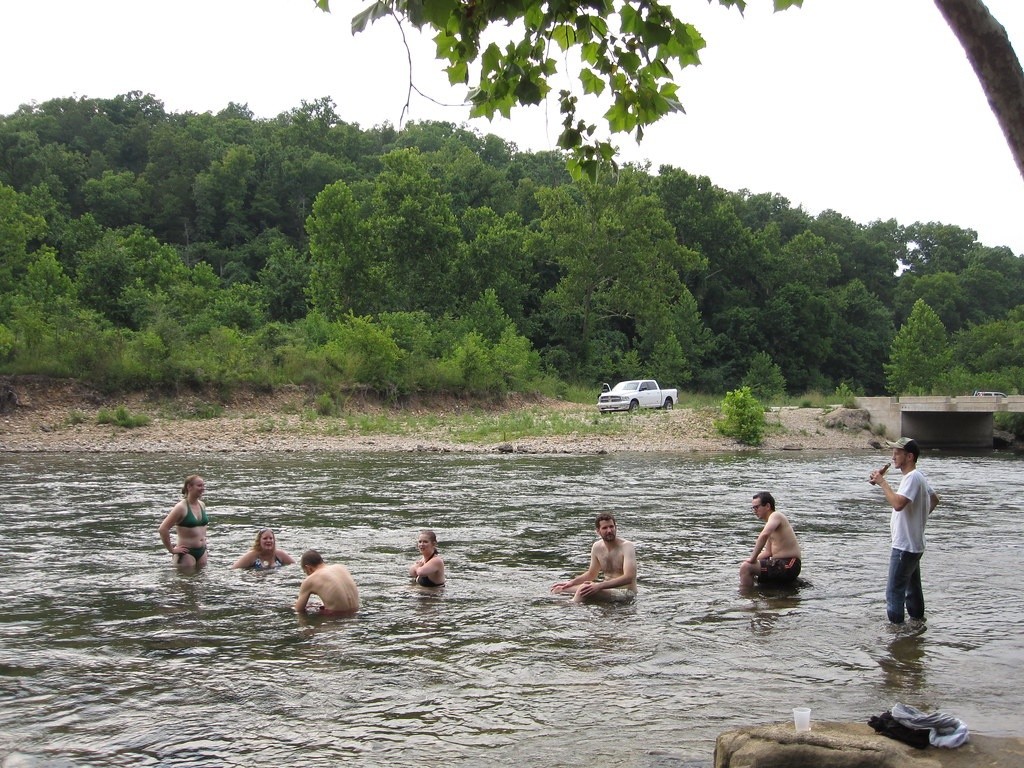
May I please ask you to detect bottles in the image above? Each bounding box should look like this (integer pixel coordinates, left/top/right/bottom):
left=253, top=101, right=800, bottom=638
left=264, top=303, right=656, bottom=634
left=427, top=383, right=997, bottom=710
left=869, top=463, right=891, bottom=486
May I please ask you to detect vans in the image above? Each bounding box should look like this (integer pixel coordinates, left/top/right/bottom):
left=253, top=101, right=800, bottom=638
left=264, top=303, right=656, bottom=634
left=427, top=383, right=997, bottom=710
left=977, top=392, right=1007, bottom=398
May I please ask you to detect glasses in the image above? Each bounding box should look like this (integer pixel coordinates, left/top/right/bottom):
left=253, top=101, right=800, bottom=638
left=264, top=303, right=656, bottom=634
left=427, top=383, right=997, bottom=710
left=259, top=528, right=273, bottom=534
left=751, top=504, right=762, bottom=511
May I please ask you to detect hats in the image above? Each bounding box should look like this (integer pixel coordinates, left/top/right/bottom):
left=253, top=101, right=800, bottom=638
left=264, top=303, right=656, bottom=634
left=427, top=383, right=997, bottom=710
left=886, top=437, right=920, bottom=456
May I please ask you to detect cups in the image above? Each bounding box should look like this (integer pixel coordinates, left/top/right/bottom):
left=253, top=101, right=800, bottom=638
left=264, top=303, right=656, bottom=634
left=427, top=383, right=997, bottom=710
left=793, top=707, right=812, bottom=732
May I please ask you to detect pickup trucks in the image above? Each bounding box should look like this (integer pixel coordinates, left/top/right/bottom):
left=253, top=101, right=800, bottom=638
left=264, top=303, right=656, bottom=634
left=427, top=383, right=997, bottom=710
left=597, top=379, right=679, bottom=416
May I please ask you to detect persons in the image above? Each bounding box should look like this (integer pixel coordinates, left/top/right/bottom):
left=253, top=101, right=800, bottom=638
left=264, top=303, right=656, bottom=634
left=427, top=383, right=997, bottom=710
left=739, top=491, right=801, bottom=587
left=409, top=529, right=447, bottom=588
left=231, top=527, right=296, bottom=570
left=158, top=475, right=210, bottom=566
left=551, top=513, right=638, bottom=603
left=294, top=548, right=359, bottom=612
left=867, top=437, right=940, bottom=625
